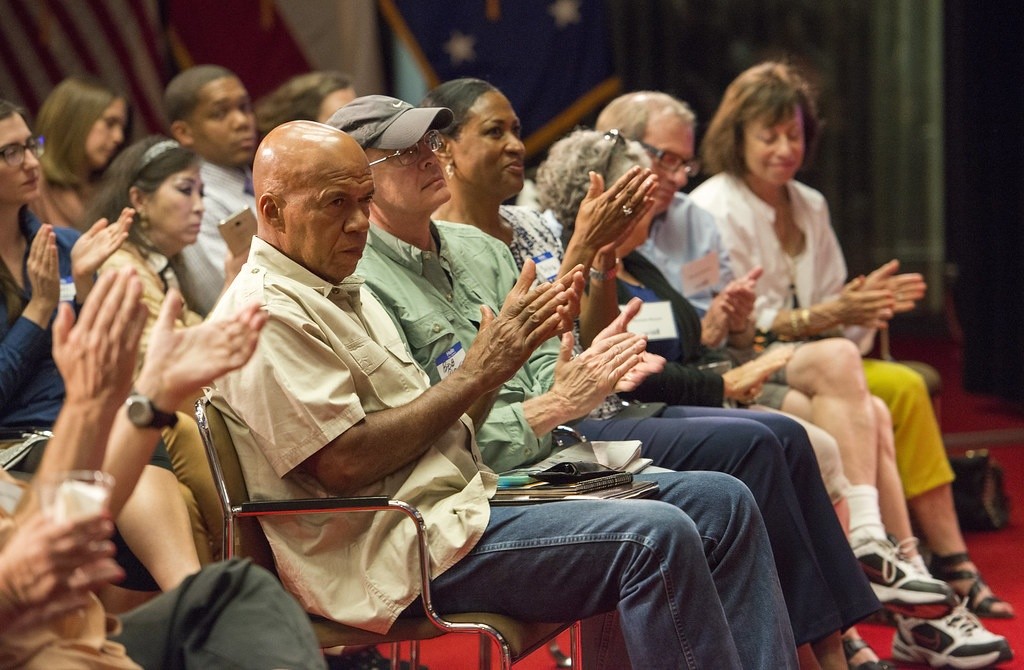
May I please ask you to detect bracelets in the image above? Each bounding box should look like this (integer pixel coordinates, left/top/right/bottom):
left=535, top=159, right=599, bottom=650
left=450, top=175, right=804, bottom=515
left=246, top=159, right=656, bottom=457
left=802, top=308, right=811, bottom=339
left=790, top=309, right=799, bottom=338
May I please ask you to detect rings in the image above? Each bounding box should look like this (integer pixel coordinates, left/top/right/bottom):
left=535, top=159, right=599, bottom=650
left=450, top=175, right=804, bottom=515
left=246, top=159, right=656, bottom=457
left=623, top=205, right=633, bottom=217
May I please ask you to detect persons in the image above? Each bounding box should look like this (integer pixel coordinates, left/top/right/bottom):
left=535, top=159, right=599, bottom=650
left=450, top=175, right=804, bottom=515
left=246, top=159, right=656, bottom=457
left=0, top=60, right=1015, bottom=670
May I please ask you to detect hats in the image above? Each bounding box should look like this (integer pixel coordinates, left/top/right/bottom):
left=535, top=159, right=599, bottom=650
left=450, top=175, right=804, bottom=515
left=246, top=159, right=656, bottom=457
left=326, top=94, right=453, bottom=153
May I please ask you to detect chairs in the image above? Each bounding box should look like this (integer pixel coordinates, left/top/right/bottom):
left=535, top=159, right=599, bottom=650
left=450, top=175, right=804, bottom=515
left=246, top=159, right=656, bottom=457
left=193, top=393, right=576, bottom=670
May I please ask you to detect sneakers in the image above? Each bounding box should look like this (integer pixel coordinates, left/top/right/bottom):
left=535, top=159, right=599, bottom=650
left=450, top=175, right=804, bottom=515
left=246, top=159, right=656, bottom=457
left=851, top=536, right=951, bottom=604
left=893, top=597, right=1013, bottom=669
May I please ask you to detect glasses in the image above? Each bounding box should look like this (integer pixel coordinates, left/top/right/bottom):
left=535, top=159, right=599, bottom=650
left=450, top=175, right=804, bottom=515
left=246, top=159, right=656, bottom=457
left=0, top=134, right=46, bottom=167
left=641, top=142, right=703, bottom=178
left=603, top=129, right=626, bottom=178
left=369, top=129, right=443, bottom=167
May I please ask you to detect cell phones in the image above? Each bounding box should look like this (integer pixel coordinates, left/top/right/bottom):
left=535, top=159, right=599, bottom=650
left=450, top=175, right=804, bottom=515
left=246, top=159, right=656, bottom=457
left=218, top=205, right=258, bottom=265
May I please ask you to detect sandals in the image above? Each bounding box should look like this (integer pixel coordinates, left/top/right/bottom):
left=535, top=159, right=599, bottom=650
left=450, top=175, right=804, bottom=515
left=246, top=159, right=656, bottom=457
left=845, top=639, right=895, bottom=670
left=929, top=552, right=1014, bottom=619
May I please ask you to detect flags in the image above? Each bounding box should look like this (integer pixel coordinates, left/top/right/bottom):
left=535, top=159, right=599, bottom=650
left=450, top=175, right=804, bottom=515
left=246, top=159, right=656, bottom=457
left=379, top=0, right=619, bottom=161
left=0, top=0, right=313, bottom=145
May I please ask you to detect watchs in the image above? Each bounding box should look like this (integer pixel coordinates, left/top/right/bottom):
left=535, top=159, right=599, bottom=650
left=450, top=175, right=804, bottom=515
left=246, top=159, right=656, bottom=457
left=588, top=258, right=619, bottom=282
left=124, top=393, right=179, bottom=429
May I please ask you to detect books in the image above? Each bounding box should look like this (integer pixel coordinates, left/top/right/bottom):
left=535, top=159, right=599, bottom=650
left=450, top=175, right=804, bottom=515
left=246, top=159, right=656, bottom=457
left=495, top=472, right=633, bottom=496
left=487, top=480, right=660, bottom=506
